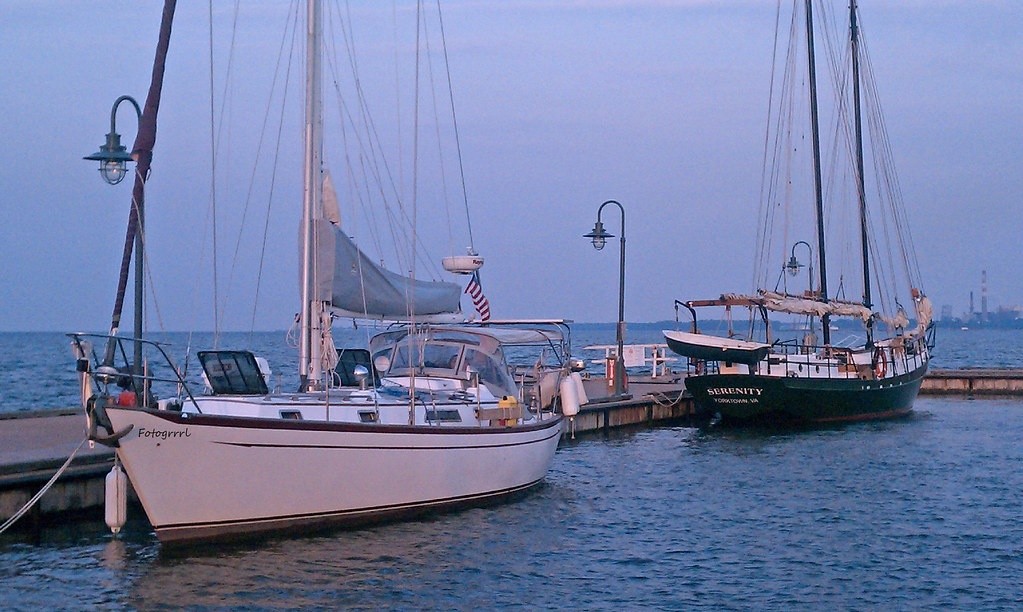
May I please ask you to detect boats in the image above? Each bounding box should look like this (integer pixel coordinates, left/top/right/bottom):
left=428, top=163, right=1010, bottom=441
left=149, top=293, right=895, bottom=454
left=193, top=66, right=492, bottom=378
left=662, top=330, right=772, bottom=365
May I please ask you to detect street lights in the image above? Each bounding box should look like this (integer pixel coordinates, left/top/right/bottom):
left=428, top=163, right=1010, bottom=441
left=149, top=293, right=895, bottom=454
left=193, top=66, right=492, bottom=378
left=583, top=200, right=632, bottom=401
left=83, top=96, right=143, bottom=386
left=787, top=240, right=815, bottom=333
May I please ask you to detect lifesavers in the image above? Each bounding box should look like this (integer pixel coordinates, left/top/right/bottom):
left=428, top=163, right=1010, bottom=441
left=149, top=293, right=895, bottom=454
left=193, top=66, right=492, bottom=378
left=874, top=350, right=887, bottom=379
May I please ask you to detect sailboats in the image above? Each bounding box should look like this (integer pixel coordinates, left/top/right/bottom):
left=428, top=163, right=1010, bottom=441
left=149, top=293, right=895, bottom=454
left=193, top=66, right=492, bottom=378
left=68, top=0, right=585, bottom=546
left=685, top=1, right=938, bottom=426
